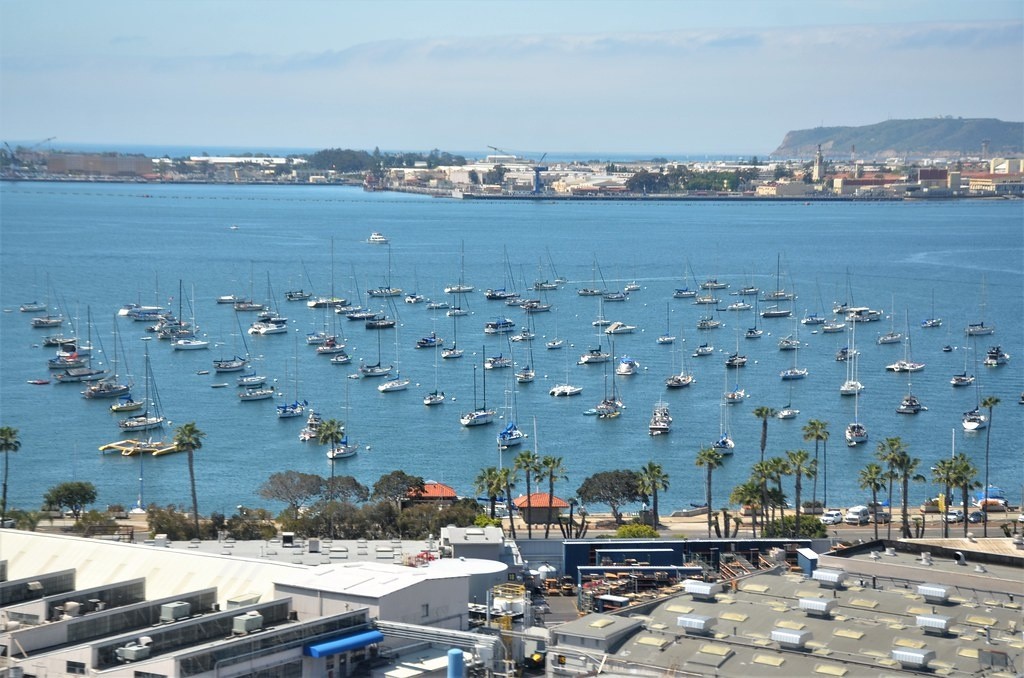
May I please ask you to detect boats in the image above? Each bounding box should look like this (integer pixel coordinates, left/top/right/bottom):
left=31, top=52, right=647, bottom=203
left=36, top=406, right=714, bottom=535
left=365, top=232, right=389, bottom=245
left=230, top=224, right=239, bottom=230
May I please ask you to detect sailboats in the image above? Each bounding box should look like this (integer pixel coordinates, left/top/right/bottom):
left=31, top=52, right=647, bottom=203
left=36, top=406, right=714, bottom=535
left=18, top=236, right=1010, bottom=461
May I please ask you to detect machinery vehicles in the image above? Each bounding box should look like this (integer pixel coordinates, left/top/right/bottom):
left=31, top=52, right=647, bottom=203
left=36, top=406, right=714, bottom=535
left=545, top=575, right=575, bottom=597
